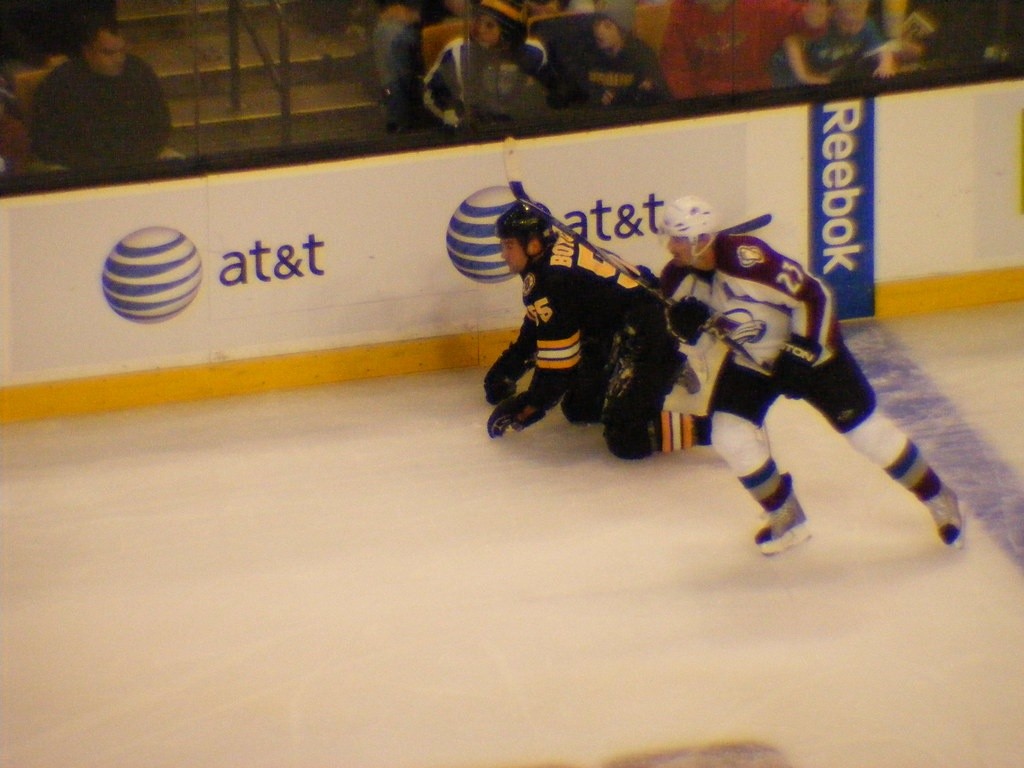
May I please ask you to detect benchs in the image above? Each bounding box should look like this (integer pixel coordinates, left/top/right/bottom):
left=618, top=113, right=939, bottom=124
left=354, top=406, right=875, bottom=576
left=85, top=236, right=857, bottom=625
left=0, top=0, right=391, bottom=193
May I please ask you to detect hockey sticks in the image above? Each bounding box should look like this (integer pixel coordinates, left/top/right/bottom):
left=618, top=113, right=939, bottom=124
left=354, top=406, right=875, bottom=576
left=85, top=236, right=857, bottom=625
left=500, top=133, right=775, bottom=372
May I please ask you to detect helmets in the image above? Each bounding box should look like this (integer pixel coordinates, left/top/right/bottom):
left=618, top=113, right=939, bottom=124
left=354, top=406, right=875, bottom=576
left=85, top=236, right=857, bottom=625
left=662, top=195, right=719, bottom=238
left=495, top=201, right=554, bottom=244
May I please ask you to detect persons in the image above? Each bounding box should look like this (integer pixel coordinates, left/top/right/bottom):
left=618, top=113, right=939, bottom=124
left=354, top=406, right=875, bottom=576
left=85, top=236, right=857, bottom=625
left=29, top=18, right=171, bottom=184
left=371, top=0, right=547, bottom=146
left=660, top=195, right=962, bottom=557
left=565, top=0, right=674, bottom=106
left=483, top=202, right=712, bottom=460
left=767, top=0, right=928, bottom=88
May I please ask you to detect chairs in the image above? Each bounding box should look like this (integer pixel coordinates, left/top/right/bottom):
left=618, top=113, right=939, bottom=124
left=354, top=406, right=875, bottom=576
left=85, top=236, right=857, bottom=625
left=633, top=0, right=887, bottom=85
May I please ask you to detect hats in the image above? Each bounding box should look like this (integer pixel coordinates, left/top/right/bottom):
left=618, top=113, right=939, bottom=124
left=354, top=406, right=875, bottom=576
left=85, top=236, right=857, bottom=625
left=478, top=0, right=526, bottom=32
left=593, top=0, right=634, bottom=34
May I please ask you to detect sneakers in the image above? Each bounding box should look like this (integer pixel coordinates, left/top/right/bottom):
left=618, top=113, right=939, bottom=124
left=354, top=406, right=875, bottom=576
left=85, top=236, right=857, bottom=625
left=910, top=470, right=964, bottom=551
left=757, top=471, right=812, bottom=555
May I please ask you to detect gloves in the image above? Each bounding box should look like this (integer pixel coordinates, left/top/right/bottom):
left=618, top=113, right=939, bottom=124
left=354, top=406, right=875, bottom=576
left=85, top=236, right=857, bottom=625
left=774, top=331, right=823, bottom=394
left=484, top=343, right=540, bottom=402
left=668, top=296, right=711, bottom=342
left=487, top=390, right=542, bottom=438
